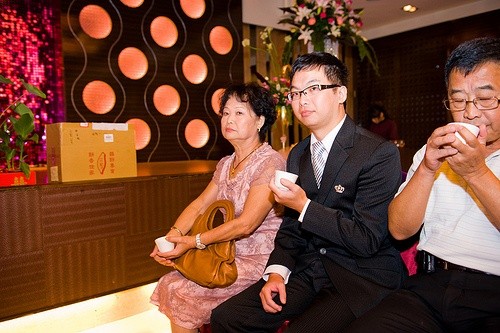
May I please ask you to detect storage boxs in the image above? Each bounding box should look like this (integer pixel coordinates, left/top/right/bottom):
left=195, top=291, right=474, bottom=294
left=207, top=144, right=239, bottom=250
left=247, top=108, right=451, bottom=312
left=44, top=122, right=137, bottom=182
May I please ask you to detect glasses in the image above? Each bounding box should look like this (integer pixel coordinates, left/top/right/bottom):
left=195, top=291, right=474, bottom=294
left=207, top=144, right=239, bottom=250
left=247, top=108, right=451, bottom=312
left=443, top=93, right=499, bottom=111
left=287, top=84, right=341, bottom=101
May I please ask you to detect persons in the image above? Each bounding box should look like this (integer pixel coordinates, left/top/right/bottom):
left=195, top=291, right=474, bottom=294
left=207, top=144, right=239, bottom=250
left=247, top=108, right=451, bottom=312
left=209, top=52, right=403, bottom=333
left=366, top=104, right=399, bottom=140
left=347, top=37, right=500, bottom=333
left=149, top=81, right=286, bottom=333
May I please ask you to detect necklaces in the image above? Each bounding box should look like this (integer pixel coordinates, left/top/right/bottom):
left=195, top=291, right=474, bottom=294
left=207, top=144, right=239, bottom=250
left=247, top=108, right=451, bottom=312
left=231, top=143, right=264, bottom=177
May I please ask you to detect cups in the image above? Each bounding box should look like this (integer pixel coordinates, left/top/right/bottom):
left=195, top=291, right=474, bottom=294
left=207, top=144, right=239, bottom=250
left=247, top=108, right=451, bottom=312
left=274, top=169, right=298, bottom=193
left=447, top=123, right=480, bottom=147
left=155, top=236, right=174, bottom=254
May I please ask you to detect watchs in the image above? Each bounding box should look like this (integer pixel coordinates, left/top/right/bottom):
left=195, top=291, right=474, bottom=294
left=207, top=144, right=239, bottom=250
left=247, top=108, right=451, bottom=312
left=196, top=233, right=206, bottom=250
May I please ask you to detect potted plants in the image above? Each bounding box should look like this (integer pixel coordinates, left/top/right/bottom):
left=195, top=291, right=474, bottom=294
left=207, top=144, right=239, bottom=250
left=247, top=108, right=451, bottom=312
left=0, top=74, right=47, bottom=186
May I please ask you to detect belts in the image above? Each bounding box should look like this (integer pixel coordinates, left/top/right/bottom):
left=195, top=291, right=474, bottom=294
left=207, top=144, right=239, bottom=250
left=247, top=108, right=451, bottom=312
left=417, top=251, right=482, bottom=274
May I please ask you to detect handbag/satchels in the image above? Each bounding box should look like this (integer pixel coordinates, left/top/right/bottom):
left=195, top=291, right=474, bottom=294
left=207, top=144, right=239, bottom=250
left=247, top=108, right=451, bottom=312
left=173, top=200, right=240, bottom=289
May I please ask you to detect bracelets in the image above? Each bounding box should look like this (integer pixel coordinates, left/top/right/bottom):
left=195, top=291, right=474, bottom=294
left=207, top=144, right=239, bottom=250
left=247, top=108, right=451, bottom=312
left=170, top=226, right=184, bottom=236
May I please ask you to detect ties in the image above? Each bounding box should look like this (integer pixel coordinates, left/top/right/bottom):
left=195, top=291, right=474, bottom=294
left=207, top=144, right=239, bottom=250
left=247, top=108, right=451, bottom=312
left=310, top=142, right=329, bottom=189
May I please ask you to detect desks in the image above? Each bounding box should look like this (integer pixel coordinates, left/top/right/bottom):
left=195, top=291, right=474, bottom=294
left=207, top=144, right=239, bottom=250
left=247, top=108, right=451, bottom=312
left=0, top=159, right=226, bottom=322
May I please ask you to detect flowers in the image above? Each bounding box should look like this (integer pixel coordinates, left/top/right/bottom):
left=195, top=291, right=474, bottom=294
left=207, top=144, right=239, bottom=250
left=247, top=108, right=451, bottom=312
left=242, top=0, right=381, bottom=107
